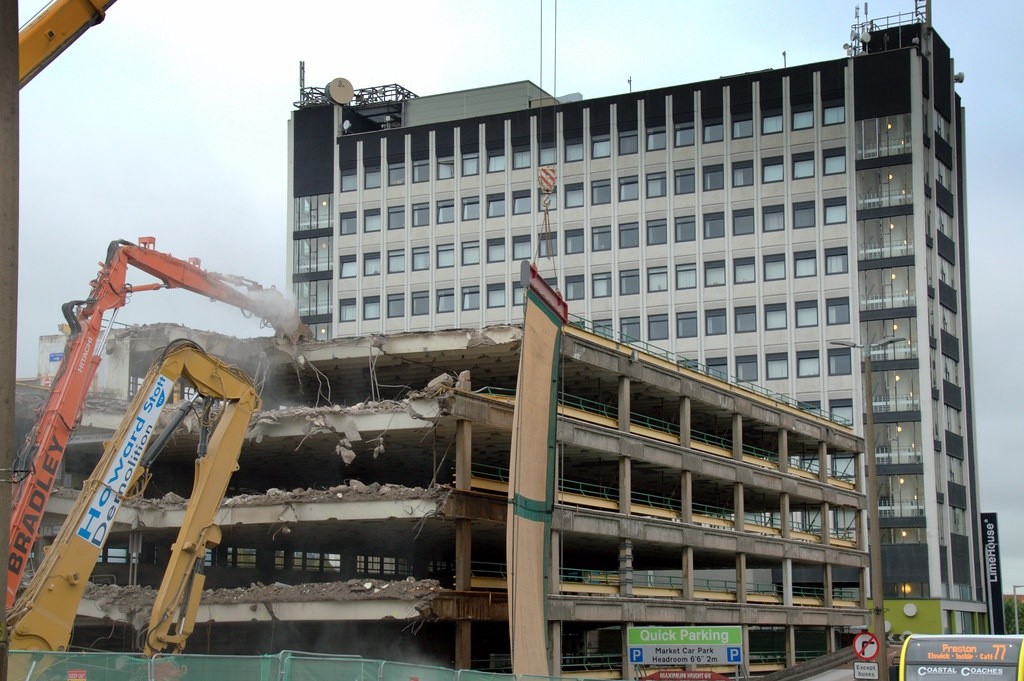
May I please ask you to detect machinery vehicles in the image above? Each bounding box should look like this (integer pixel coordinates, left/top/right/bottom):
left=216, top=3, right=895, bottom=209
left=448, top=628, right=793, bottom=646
left=6, top=238, right=315, bottom=665
left=20, top=338, right=258, bottom=660
left=19, top=2, right=118, bottom=90
